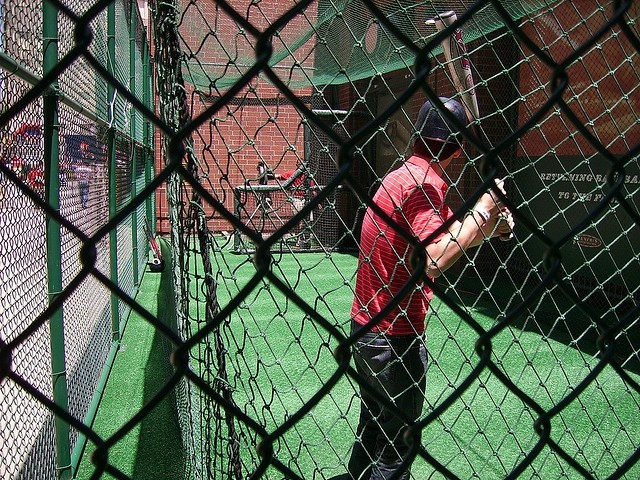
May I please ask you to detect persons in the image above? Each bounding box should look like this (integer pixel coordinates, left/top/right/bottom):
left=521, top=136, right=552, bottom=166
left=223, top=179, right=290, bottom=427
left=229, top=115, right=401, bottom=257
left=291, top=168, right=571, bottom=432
left=26, top=160, right=45, bottom=209
left=274, top=158, right=323, bottom=241
left=347, top=97, right=516, bottom=479
left=75, top=161, right=92, bottom=210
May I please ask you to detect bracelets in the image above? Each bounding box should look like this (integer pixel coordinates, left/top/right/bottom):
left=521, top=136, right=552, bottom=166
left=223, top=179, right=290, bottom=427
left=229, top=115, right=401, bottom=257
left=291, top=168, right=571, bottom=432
left=474, top=204, right=490, bottom=223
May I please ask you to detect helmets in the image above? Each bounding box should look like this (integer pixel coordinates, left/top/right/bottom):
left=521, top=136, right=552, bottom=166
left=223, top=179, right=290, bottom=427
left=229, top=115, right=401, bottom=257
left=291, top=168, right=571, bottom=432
left=410, top=97, right=466, bottom=144
left=146, top=259, right=162, bottom=272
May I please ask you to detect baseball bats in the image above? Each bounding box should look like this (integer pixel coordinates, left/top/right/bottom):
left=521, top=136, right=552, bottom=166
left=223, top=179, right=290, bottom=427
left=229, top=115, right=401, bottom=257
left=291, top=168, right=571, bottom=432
left=143, top=216, right=162, bottom=263
left=434, top=11, right=513, bottom=241
